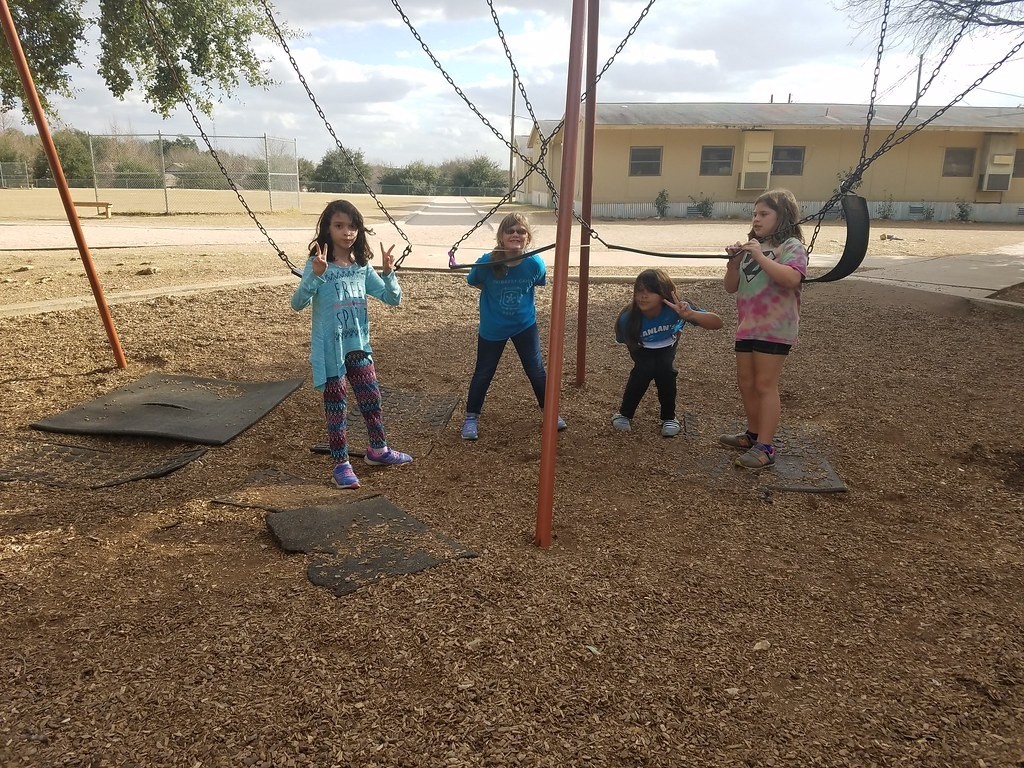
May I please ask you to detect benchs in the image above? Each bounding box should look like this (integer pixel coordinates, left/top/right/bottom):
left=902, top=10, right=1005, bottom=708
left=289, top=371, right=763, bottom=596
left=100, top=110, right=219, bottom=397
left=73, top=202, right=112, bottom=218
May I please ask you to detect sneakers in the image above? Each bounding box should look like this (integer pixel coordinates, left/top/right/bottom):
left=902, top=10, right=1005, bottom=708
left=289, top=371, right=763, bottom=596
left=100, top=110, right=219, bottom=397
left=735, top=445, right=775, bottom=470
left=718, top=432, right=756, bottom=450
left=461, top=417, right=478, bottom=439
left=557, top=416, right=567, bottom=431
left=331, top=463, right=360, bottom=489
left=611, top=414, right=631, bottom=432
left=363, top=446, right=413, bottom=465
left=661, top=417, right=681, bottom=436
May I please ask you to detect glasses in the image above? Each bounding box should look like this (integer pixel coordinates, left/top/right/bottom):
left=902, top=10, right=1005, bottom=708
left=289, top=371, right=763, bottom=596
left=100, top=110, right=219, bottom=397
left=504, top=227, right=528, bottom=235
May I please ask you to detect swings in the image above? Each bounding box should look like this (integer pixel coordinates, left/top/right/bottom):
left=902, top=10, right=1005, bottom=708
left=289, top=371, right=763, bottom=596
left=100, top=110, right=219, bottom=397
left=731, top=0, right=1024, bottom=286
left=390, top=0, right=730, bottom=271
left=139, top=0, right=415, bottom=282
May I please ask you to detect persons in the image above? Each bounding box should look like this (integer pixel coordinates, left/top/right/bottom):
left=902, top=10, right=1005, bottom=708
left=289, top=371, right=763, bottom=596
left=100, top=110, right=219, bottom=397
left=718, top=188, right=807, bottom=469
left=461, top=212, right=565, bottom=438
left=611, top=269, right=722, bottom=438
left=291, top=199, right=413, bottom=488
